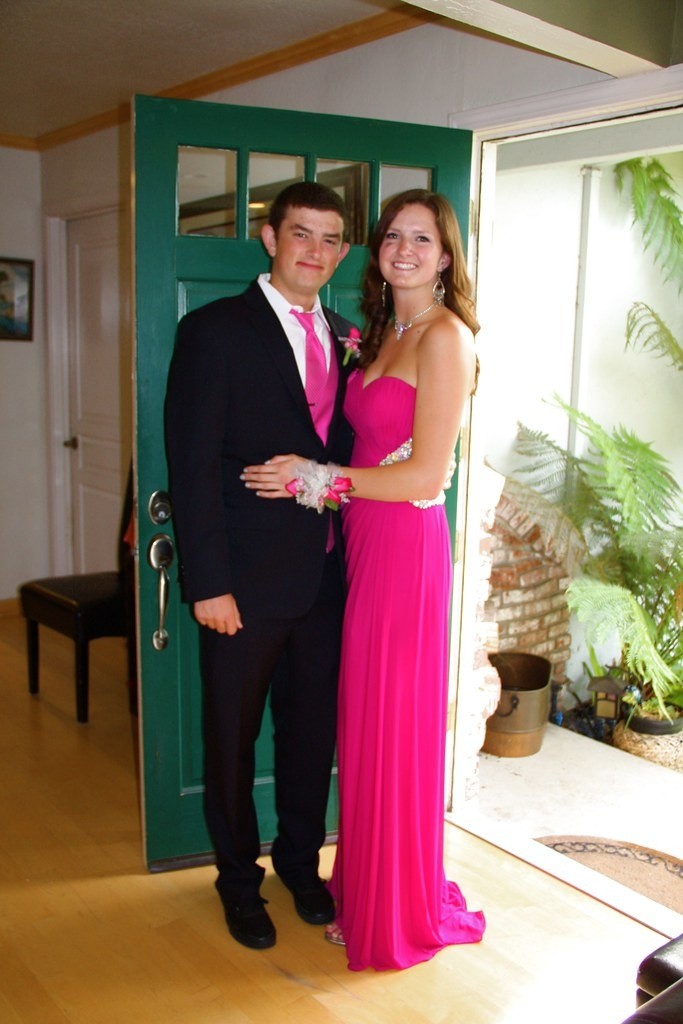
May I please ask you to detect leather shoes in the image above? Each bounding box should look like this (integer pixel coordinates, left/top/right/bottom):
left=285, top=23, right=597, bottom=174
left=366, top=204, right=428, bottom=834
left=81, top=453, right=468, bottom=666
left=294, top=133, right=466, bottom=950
left=214, top=879, right=277, bottom=949
left=273, top=862, right=335, bottom=925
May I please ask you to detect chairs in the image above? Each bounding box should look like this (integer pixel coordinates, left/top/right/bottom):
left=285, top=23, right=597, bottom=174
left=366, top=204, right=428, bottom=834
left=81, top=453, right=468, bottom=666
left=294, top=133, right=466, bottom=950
left=19, top=452, right=135, bottom=723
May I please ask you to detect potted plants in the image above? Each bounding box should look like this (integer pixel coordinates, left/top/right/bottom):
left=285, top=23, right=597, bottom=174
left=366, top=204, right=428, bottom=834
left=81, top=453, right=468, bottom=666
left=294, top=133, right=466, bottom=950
left=486, top=393, right=683, bottom=775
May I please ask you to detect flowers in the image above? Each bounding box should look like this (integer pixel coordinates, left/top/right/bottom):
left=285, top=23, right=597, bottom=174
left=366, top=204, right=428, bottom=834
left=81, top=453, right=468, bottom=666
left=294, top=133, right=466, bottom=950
left=338, top=328, right=362, bottom=366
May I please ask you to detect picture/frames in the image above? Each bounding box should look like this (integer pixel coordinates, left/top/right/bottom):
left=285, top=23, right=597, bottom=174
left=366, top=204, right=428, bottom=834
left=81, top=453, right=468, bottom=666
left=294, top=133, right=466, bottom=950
left=0, top=255, right=36, bottom=342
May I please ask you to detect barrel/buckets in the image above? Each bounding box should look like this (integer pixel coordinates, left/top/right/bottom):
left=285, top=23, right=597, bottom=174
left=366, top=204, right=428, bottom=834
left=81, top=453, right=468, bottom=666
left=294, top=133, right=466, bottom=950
left=479, top=651, right=553, bottom=758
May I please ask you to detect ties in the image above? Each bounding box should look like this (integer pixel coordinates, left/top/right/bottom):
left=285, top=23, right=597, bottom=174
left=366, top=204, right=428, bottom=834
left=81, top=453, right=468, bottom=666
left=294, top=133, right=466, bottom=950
left=290, top=309, right=335, bottom=554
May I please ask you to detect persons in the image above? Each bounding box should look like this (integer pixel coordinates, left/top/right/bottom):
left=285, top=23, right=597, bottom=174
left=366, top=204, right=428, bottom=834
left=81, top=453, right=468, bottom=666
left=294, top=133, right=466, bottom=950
left=160, top=180, right=458, bottom=950
left=239, top=189, right=487, bottom=972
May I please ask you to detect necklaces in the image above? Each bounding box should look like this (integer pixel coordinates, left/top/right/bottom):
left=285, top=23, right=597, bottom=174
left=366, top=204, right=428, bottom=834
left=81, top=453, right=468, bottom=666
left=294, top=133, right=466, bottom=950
left=392, top=301, right=438, bottom=341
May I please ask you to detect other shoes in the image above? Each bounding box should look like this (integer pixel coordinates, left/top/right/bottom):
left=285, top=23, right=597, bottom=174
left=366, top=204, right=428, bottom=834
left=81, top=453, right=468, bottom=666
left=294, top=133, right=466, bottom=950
left=326, top=920, right=346, bottom=945
